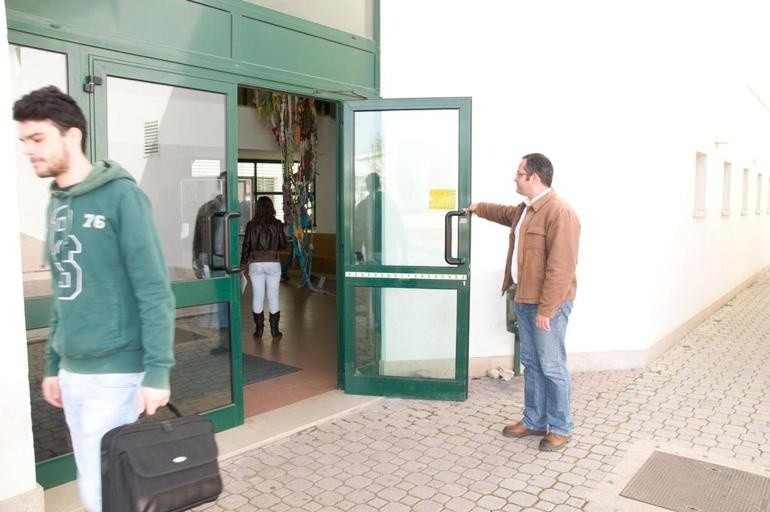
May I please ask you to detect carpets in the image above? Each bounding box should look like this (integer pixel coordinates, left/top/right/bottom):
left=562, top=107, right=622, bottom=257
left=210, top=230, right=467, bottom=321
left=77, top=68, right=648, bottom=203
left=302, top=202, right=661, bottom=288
left=174, top=328, right=208, bottom=344
left=169, top=350, right=304, bottom=406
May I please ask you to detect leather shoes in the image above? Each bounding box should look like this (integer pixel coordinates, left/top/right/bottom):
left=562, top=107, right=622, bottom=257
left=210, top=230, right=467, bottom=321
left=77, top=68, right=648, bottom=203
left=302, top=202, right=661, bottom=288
left=503, top=420, right=547, bottom=438
left=538, top=432, right=574, bottom=451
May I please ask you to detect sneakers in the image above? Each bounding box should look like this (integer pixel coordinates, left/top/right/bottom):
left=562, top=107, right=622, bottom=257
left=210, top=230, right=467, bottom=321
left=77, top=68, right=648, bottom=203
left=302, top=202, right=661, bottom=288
left=210, top=345, right=227, bottom=355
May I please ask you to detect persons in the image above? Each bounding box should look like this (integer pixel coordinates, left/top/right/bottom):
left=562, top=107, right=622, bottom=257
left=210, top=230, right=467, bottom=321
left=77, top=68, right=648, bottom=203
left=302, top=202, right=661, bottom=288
left=10, top=84, right=178, bottom=512
left=241, top=195, right=289, bottom=337
left=461, top=152, right=583, bottom=454
left=190, top=171, right=231, bottom=356
left=354, top=171, right=397, bottom=333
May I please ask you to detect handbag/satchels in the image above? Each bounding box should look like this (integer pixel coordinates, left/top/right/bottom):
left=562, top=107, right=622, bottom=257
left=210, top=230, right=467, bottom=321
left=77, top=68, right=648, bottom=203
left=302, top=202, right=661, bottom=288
left=101, top=402, right=223, bottom=512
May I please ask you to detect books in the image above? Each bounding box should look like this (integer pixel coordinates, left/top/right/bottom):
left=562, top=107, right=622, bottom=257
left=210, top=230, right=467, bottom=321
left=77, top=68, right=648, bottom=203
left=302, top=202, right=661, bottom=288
left=241, top=272, right=248, bottom=296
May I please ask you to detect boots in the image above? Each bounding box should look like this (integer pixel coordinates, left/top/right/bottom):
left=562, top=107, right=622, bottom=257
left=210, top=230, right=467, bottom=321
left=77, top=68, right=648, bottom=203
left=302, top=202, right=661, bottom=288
left=269, top=311, right=282, bottom=337
left=253, top=311, right=264, bottom=338
